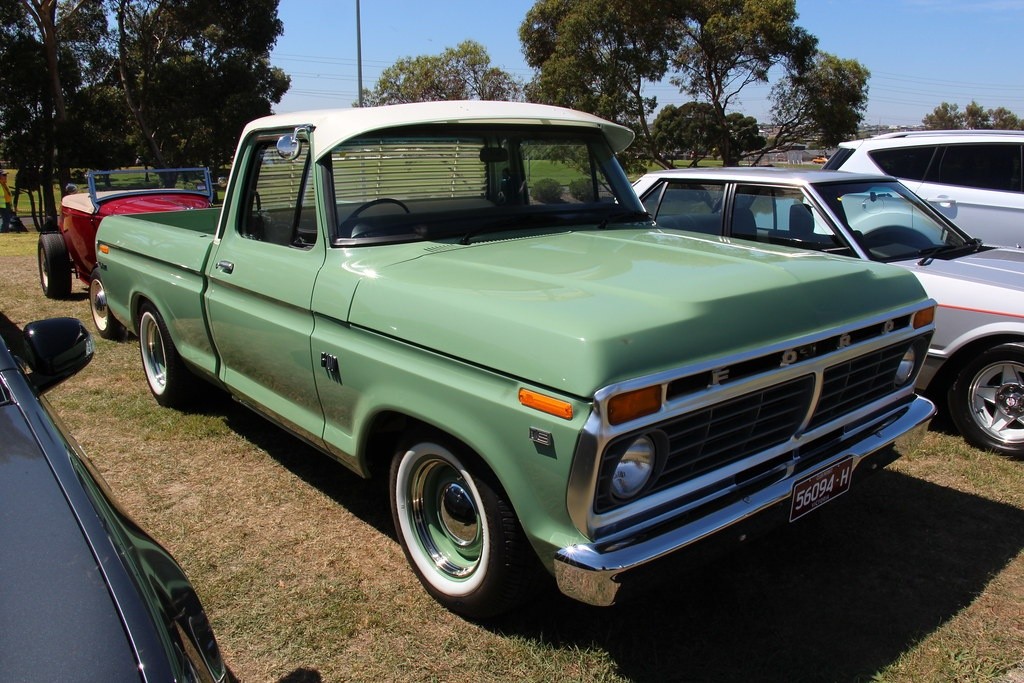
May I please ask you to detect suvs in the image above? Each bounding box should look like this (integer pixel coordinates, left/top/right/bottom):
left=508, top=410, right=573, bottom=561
left=799, top=129, right=1024, bottom=246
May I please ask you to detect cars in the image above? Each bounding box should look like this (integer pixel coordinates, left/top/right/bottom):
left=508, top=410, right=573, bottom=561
left=633, top=166, right=1024, bottom=462
left=1, top=310, right=235, bottom=683
left=36, top=166, right=215, bottom=300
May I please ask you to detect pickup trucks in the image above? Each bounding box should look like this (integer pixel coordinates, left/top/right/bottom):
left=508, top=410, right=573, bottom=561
left=93, top=98, right=936, bottom=621
